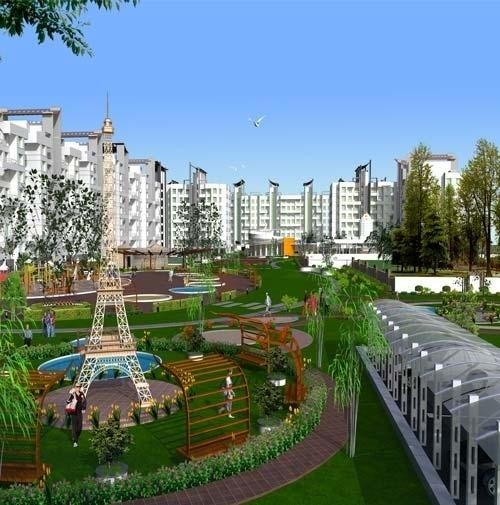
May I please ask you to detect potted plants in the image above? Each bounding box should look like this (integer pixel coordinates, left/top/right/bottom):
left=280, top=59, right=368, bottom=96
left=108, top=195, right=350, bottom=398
left=252, top=346, right=289, bottom=435
left=87, top=418, right=135, bottom=485
left=184, top=328, right=206, bottom=362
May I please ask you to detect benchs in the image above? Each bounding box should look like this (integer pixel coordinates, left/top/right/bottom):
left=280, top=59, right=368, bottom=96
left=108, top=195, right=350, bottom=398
left=177, top=429, right=253, bottom=464
left=0, top=461, right=51, bottom=492
left=234, top=352, right=272, bottom=374
left=283, top=383, right=307, bottom=414
